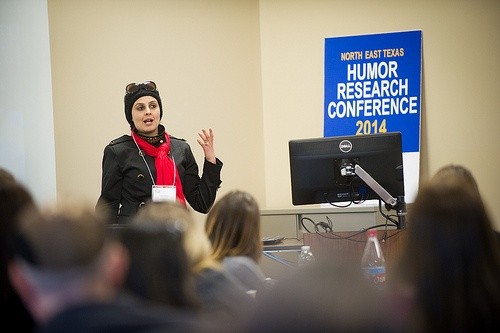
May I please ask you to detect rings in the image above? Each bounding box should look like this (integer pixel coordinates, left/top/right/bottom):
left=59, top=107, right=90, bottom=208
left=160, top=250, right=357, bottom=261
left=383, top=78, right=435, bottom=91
left=204, top=139, right=208, bottom=143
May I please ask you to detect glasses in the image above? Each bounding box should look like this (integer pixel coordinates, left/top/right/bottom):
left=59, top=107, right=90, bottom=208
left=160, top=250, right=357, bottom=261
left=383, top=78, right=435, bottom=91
left=125, top=81, right=157, bottom=94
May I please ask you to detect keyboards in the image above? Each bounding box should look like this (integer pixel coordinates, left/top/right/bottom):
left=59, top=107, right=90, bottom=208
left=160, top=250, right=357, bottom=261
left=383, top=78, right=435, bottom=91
left=262, top=236, right=285, bottom=245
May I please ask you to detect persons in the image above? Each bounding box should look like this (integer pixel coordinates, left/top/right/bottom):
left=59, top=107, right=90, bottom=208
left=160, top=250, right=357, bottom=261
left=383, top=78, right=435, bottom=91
left=0, top=164, right=500, bottom=333
left=94, top=80, right=224, bottom=229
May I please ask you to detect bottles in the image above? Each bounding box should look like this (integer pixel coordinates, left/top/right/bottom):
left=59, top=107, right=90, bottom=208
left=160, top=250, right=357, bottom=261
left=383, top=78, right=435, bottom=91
left=360, top=229, right=386, bottom=285
left=297, top=244, right=314, bottom=267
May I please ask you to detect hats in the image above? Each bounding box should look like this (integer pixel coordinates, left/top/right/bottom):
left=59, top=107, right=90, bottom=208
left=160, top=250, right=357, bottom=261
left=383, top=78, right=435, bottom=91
left=124, top=84, right=162, bottom=125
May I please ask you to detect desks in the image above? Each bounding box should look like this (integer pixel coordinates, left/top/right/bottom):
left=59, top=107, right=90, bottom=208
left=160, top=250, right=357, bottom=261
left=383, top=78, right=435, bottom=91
left=261, top=238, right=304, bottom=270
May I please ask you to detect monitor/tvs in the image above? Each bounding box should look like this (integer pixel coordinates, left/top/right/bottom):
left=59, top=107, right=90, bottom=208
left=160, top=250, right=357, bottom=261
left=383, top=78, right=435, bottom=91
left=289, top=131, right=405, bottom=206
